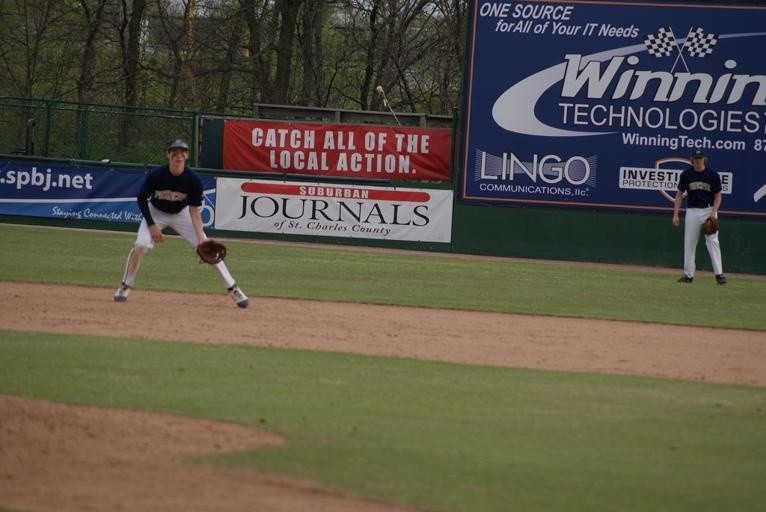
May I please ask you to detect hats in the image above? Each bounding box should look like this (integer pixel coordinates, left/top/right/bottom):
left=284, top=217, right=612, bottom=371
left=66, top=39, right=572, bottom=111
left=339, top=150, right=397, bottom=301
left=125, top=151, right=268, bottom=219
left=691, top=149, right=705, bottom=160
left=167, top=138, right=190, bottom=153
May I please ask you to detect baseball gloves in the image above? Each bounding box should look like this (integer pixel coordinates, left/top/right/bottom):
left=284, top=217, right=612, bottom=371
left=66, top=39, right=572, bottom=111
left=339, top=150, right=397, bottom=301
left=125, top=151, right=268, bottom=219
left=703, top=216, right=719, bottom=234
left=196, top=241, right=225, bottom=265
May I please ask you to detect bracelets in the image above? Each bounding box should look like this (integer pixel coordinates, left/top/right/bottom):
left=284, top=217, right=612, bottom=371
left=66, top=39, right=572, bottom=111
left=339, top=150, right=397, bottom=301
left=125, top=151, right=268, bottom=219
left=673, top=214, right=678, bottom=216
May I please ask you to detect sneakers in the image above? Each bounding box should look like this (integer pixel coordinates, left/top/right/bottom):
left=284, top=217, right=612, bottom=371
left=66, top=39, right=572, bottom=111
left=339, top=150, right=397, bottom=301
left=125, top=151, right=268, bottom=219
left=225, top=284, right=250, bottom=309
left=113, top=285, right=129, bottom=303
left=677, top=274, right=694, bottom=283
left=715, top=274, right=727, bottom=286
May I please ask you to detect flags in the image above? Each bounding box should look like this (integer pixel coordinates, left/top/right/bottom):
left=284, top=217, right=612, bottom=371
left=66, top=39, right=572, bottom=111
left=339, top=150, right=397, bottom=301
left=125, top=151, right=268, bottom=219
left=683, top=27, right=719, bottom=59
left=644, top=26, right=678, bottom=58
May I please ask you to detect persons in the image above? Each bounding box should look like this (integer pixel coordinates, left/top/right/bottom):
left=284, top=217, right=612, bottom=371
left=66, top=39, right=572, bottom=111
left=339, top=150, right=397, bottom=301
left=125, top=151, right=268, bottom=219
left=672, top=148, right=727, bottom=284
left=113, top=138, right=249, bottom=307
